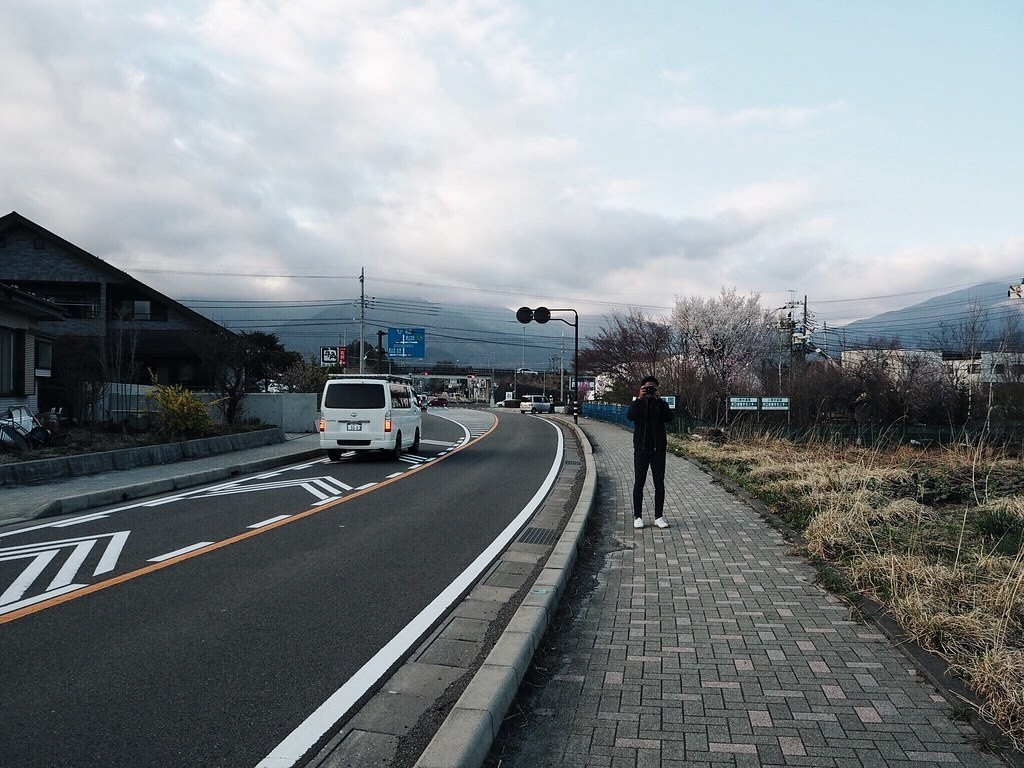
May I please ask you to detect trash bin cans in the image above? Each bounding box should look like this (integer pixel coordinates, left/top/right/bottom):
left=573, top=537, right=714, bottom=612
left=129, top=413, right=149, bottom=432
left=46, top=412, right=61, bottom=434
left=35, top=413, right=47, bottom=427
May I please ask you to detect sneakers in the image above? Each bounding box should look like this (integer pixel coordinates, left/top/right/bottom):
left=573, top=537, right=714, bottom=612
left=654, top=516, right=667, bottom=528
left=634, top=517, right=643, bottom=528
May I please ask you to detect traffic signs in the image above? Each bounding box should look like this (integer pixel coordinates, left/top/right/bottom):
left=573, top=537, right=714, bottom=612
left=387, top=327, right=425, bottom=375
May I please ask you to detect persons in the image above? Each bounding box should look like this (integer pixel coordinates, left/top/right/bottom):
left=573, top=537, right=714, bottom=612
left=626, top=376, right=674, bottom=528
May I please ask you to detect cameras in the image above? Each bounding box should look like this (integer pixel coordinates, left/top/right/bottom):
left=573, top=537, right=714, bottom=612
left=645, top=385, right=657, bottom=394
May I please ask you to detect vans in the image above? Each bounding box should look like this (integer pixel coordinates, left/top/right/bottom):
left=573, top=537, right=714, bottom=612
left=320, top=378, right=426, bottom=462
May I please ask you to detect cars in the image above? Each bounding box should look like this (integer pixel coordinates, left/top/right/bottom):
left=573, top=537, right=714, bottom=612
left=428, top=398, right=450, bottom=407
left=496, top=398, right=517, bottom=408
left=520, top=395, right=552, bottom=414
left=517, top=368, right=539, bottom=376
